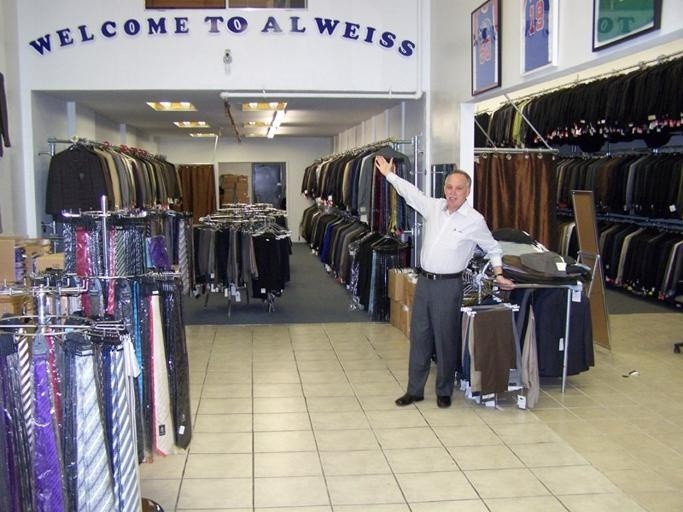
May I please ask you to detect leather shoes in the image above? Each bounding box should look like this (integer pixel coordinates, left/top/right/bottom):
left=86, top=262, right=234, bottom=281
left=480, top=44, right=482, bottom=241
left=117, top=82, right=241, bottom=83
left=396, top=393, right=423, bottom=405
left=437, top=396, right=450, bottom=407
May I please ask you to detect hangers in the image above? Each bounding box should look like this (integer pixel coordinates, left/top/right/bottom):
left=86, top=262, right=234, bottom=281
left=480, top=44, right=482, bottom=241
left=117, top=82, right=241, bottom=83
left=604, top=212, right=683, bottom=235
left=68, top=134, right=168, bottom=164
left=314, top=136, right=410, bottom=251
left=193, top=202, right=293, bottom=240
left=639, top=55, right=665, bottom=71
left=459, top=267, right=521, bottom=316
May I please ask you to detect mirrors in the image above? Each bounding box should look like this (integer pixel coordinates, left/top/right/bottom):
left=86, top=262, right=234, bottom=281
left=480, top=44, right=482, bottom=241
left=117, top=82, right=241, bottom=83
left=570, top=190, right=612, bottom=350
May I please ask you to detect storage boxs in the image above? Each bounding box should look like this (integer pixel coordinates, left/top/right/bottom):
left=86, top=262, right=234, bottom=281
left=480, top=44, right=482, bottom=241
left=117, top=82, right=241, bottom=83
left=220, top=174, right=250, bottom=204
left=387, top=268, right=418, bottom=338
left=0, top=235, right=65, bottom=323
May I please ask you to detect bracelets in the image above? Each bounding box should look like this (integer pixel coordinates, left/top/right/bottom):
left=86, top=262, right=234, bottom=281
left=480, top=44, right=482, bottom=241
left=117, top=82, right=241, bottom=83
left=493, top=273, right=503, bottom=278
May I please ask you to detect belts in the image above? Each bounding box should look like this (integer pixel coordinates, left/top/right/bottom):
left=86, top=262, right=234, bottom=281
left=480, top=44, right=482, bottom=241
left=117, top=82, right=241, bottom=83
left=418, top=267, right=461, bottom=280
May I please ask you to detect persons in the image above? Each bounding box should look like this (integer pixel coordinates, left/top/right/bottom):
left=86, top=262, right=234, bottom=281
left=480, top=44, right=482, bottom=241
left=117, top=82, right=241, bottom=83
left=373, top=152, right=518, bottom=410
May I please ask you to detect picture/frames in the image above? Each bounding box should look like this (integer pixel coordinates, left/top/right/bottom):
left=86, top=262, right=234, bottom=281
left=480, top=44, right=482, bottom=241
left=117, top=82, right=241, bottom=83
left=471, top=0, right=501, bottom=96
left=593, top=1, right=661, bottom=53
left=520, top=0, right=559, bottom=77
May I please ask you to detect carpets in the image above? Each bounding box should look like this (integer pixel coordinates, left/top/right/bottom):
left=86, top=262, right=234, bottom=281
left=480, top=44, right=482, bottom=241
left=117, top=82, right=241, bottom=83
left=181, top=241, right=682, bottom=326
